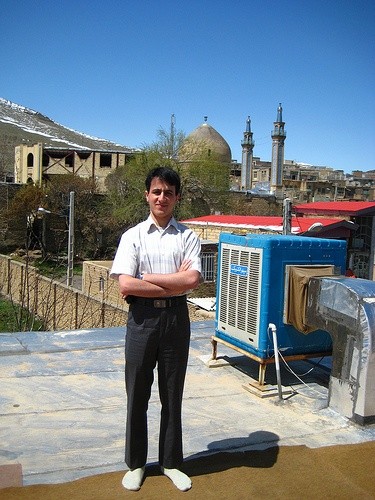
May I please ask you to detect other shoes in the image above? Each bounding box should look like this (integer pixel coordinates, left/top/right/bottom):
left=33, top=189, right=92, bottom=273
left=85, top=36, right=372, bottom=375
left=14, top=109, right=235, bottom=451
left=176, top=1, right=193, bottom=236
left=121, top=466, right=146, bottom=491
left=160, top=466, right=191, bottom=491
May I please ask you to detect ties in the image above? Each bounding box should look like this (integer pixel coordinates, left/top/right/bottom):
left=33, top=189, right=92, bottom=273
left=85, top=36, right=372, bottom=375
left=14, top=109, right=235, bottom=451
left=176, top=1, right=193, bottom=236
left=129, top=297, right=186, bottom=308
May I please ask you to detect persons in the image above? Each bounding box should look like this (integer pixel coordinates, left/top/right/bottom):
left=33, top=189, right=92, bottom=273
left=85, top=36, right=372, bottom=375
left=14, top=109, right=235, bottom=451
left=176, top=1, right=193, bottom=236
left=109, top=166, right=204, bottom=492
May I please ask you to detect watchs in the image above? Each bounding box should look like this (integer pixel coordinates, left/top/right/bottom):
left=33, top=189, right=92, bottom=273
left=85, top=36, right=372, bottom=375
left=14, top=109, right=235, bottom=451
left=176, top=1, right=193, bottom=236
left=136, top=272, right=143, bottom=279
